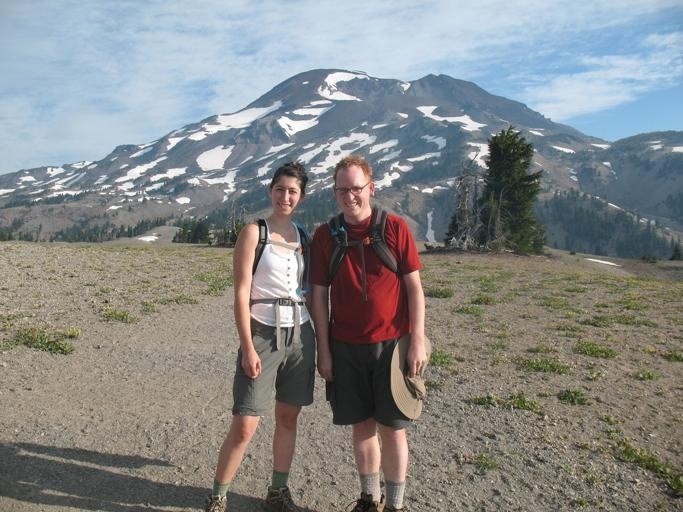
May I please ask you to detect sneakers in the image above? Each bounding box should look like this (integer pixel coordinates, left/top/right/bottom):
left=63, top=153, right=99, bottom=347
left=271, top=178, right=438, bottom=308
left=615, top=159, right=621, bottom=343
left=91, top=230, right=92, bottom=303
left=344, top=491, right=386, bottom=512
left=263, top=485, right=301, bottom=512
left=205, top=495, right=228, bottom=512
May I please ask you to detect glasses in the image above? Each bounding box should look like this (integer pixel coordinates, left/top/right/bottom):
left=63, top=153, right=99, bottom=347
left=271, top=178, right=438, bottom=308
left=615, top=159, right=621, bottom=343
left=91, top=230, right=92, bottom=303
left=334, top=182, right=371, bottom=195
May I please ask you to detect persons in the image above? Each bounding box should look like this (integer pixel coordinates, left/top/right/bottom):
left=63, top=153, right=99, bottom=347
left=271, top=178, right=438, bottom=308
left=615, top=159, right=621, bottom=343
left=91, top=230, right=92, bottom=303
left=310, top=155, right=428, bottom=512
left=203, top=160, right=315, bottom=510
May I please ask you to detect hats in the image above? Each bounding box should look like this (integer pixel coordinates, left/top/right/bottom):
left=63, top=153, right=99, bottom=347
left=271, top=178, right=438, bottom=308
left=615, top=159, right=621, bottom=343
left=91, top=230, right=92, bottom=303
left=387, top=332, right=432, bottom=420
